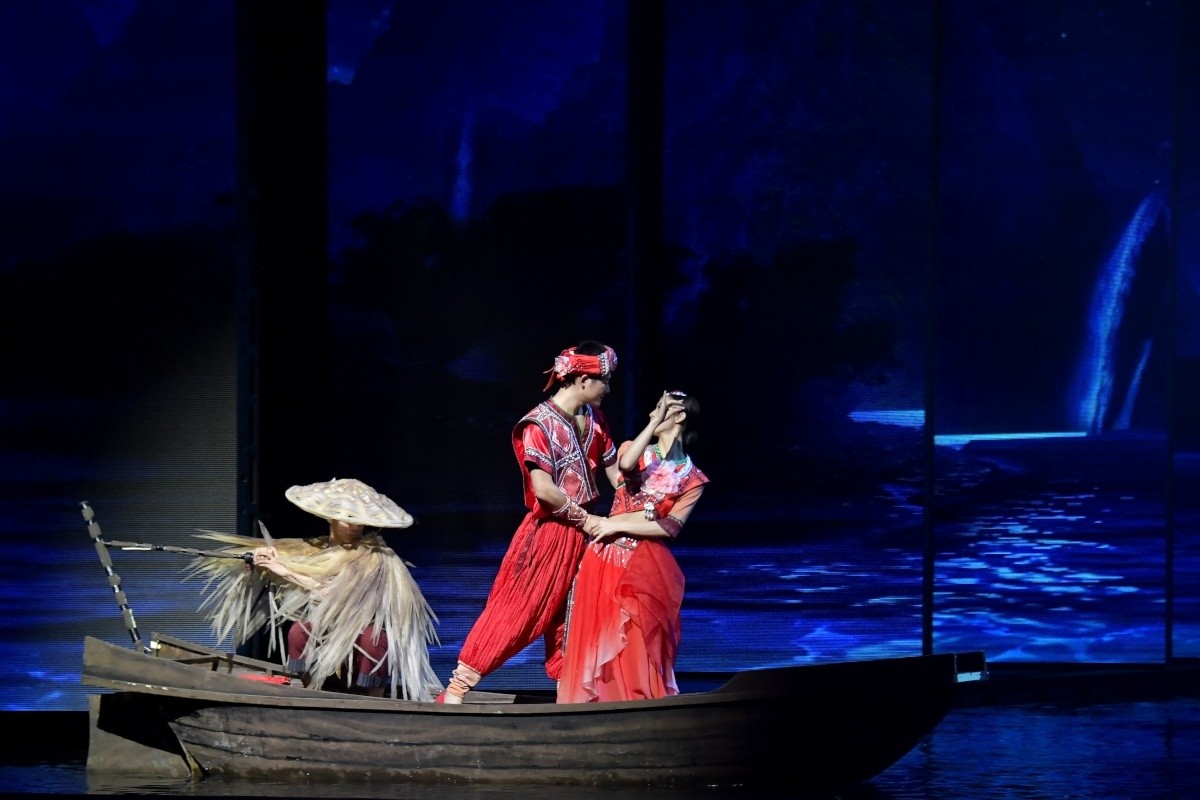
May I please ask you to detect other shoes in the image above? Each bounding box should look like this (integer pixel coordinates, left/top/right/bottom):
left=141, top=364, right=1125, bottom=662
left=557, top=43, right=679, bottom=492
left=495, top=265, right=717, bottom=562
left=296, top=671, right=311, bottom=688
left=367, top=686, right=385, bottom=697
left=436, top=689, right=462, bottom=705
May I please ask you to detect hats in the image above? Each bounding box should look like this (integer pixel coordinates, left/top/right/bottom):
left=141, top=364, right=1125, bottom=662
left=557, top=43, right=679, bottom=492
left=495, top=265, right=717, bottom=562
left=286, top=478, right=414, bottom=528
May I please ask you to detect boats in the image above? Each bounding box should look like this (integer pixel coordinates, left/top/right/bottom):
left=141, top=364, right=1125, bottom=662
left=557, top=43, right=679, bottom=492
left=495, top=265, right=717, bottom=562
left=81, top=636, right=994, bottom=799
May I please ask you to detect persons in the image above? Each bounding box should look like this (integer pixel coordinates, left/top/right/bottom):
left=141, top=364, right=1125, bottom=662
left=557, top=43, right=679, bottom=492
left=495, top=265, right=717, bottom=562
left=179, top=477, right=444, bottom=701
left=556, top=389, right=710, bottom=702
left=434, top=342, right=624, bottom=704
